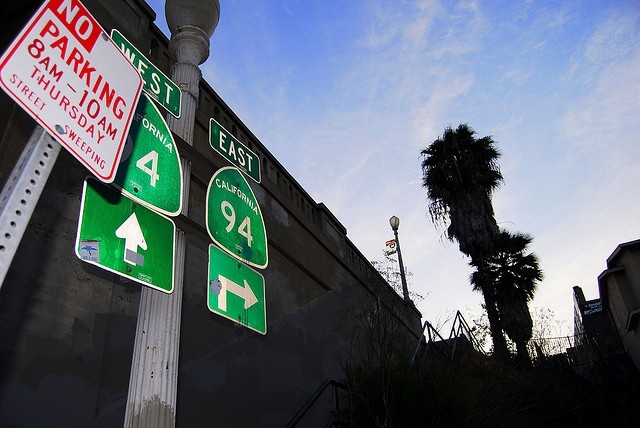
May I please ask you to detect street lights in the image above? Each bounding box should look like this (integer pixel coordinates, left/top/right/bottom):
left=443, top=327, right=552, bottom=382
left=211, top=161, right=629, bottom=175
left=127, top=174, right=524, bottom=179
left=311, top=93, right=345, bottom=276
left=389, top=216, right=411, bottom=302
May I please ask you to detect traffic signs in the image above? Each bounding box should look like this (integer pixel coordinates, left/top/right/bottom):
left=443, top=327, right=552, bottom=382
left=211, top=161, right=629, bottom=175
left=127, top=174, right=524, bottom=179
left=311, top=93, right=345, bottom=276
left=206, top=167, right=267, bottom=337
left=71, top=87, right=184, bottom=294
left=105, top=30, right=182, bottom=119
left=209, top=117, right=261, bottom=184
